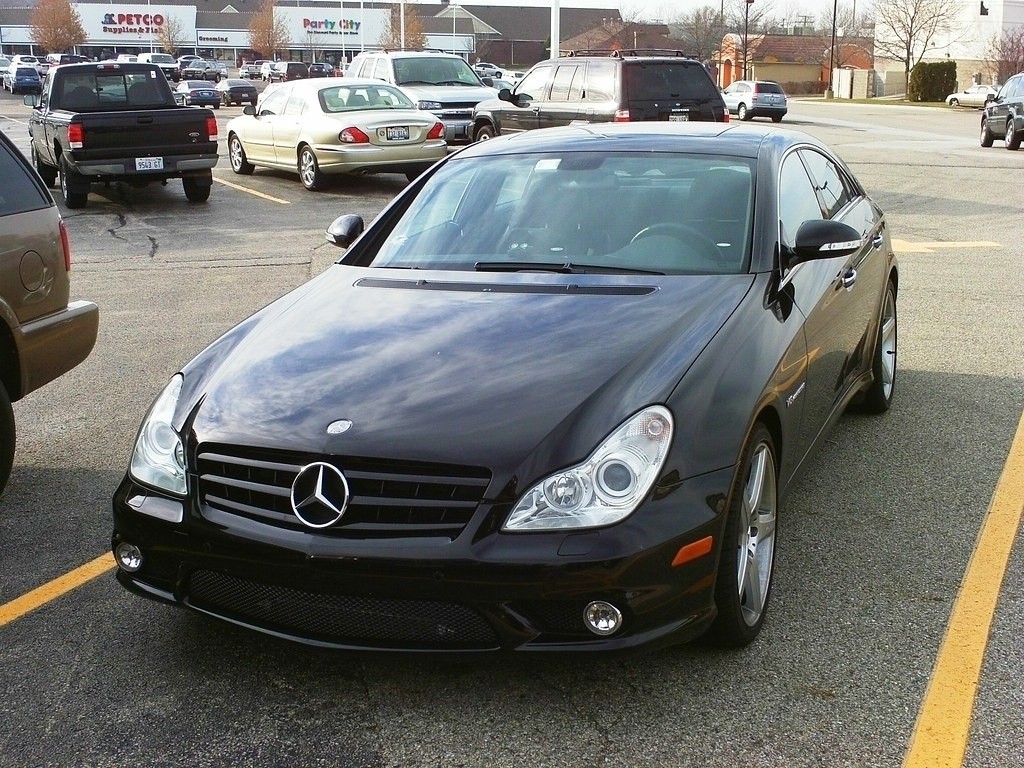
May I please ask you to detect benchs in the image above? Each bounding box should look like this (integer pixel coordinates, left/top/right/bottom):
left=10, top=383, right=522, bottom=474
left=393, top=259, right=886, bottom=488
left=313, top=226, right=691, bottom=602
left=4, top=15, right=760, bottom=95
left=617, top=177, right=694, bottom=247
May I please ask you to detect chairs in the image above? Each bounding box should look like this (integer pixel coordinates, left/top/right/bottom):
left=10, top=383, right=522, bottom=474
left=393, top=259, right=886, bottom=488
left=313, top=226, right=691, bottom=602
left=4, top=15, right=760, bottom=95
left=127, top=83, right=152, bottom=105
left=328, top=98, right=345, bottom=107
left=71, top=86, right=97, bottom=108
left=350, top=95, right=367, bottom=105
left=374, top=96, right=393, bottom=105
left=497, top=170, right=627, bottom=257
left=684, top=169, right=751, bottom=261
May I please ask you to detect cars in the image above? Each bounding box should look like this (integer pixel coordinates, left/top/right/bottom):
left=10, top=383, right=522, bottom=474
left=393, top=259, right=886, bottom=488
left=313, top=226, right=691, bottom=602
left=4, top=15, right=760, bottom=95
left=110, top=121, right=900, bottom=662
left=171, top=80, right=223, bottom=109
left=471, top=78, right=518, bottom=95
left=471, top=63, right=508, bottom=79
left=979, top=72, right=1024, bottom=151
left=217, top=63, right=230, bottom=79
left=945, top=84, right=1008, bottom=107
left=181, top=60, right=222, bottom=83
left=226, top=77, right=448, bottom=188
left=176, top=55, right=204, bottom=63
left=1, top=130, right=101, bottom=493
left=0, top=51, right=137, bottom=94
left=501, top=71, right=530, bottom=89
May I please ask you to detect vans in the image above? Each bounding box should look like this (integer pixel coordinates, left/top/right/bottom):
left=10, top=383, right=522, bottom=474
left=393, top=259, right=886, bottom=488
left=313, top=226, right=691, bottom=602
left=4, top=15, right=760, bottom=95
left=137, top=52, right=181, bottom=82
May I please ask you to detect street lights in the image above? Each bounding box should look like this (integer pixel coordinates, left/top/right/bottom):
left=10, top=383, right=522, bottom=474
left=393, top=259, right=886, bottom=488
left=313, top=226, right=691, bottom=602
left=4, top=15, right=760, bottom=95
left=744, top=0, right=755, bottom=80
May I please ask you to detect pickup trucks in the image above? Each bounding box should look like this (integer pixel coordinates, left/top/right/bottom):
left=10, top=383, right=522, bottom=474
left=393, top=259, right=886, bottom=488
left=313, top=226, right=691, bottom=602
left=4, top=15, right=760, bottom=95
left=23, top=62, right=220, bottom=209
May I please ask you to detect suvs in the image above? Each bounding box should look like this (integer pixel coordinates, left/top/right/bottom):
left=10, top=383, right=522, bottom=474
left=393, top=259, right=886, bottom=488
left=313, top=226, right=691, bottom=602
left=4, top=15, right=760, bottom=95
left=238, top=60, right=277, bottom=81
left=719, top=79, right=788, bottom=122
left=309, top=63, right=335, bottom=78
left=467, top=48, right=730, bottom=174
left=268, top=62, right=308, bottom=84
left=336, top=48, right=504, bottom=143
